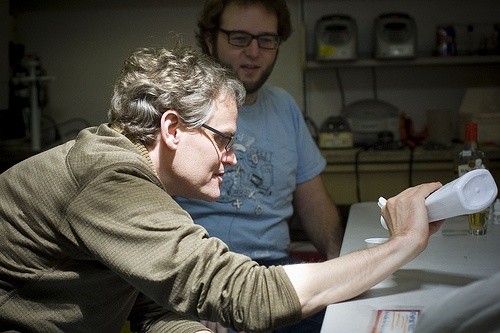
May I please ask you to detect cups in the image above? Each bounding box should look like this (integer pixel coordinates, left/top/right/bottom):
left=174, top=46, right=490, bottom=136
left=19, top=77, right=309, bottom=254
left=365, top=238, right=389, bottom=247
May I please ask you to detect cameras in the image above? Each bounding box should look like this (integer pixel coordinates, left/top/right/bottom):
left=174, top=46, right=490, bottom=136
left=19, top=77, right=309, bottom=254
left=372, top=131, right=404, bottom=150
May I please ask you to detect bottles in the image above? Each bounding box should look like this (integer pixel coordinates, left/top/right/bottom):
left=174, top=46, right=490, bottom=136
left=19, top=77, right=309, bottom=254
left=457, top=123, right=489, bottom=234
left=377, top=168, right=498, bottom=231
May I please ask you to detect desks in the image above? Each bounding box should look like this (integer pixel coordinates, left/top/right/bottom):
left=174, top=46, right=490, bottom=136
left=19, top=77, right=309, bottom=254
left=320, top=202, right=500, bottom=333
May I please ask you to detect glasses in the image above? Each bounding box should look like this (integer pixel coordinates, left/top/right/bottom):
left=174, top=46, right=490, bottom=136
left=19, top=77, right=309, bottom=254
left=194, top=120, right=237, bottom=148
left=219, top=27, right=280, bottom=48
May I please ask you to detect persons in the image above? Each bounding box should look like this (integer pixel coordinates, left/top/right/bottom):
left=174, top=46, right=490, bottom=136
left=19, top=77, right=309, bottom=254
left=0, top=35, right=444, bottom=333
left=174, top=0, right=343, bottom=333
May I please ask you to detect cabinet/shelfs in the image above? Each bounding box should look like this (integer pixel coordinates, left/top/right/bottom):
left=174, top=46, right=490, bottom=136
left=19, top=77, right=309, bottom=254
left=300, top=0, right=500, bottom=204
